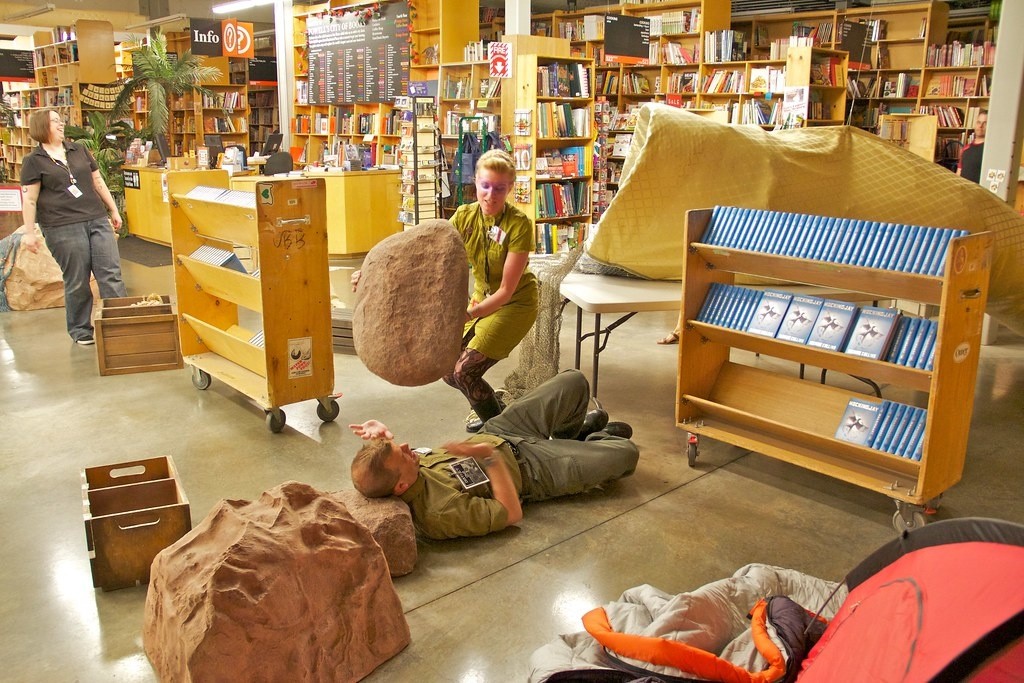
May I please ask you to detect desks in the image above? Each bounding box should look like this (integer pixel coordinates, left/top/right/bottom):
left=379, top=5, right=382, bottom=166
left=528, top=253, right=681, bottom=399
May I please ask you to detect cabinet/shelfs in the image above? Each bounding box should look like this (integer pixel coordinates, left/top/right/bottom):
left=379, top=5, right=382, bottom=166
left=0, top=0, right=1024, bottom=533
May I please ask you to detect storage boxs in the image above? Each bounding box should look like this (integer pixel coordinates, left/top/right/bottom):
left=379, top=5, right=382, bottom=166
left=95, top=296, right=184, bottom=375
left=80, top=454, right=195, bottom=593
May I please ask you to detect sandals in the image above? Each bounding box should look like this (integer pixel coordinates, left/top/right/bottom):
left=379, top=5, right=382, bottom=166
left=656, top=331, right=680, bottom=345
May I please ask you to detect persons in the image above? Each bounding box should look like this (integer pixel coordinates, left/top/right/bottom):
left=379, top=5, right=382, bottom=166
left=346, top=369, right=640, bottom=541
left=349, top=149, right=540, bottom=433
left=954, top=110, right=989, bottom=185
left=20, top=108, right=124, bottom=345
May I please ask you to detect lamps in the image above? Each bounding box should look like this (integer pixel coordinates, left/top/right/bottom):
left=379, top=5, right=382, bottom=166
left=127, top=13, right=186, bottom=31
left=212, top=0, right=275, bottom=13
left=3, top=4, right=54, bottom=22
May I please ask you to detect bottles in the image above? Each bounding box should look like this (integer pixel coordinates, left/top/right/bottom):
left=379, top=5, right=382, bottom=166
left=324, top=146, right=328, bottom=155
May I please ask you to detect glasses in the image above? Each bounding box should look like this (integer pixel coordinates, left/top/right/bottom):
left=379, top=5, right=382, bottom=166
left=49, top=118, right=65, bottom=125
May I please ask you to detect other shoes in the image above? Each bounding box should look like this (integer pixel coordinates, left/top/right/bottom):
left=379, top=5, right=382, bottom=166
left=75, top=334, right=96, bottom=345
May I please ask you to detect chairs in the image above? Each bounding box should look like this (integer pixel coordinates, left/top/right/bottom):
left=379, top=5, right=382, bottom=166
left=264, top=152, right=292, bottom=175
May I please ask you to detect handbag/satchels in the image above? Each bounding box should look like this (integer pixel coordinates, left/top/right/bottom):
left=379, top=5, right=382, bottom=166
left=449, top=129, right=504, bottom=187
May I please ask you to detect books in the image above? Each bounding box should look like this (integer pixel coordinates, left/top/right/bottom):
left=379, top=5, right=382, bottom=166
left=3, top=8, right=1000, bottom=461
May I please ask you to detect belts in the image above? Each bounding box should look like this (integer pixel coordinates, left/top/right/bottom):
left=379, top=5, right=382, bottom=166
left=476, top=430, right=531, bottom=505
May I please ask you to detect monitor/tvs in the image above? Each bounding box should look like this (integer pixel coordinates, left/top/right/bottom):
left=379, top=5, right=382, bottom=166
left=155, top=133, right=172, bottom=164
left=258, top=133, right=284, bottom=156
left=204, top=134, right=225, bottom=168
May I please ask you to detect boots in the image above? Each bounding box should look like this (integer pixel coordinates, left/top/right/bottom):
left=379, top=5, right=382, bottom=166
left=583, top=421, right=634, bottom=441
left=563, top=408, right=609, bottom=445
left=464, top=395, right=507, bottom=433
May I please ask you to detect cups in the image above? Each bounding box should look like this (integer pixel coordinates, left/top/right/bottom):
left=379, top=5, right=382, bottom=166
left=314, top=161, right=318, bottom=167
